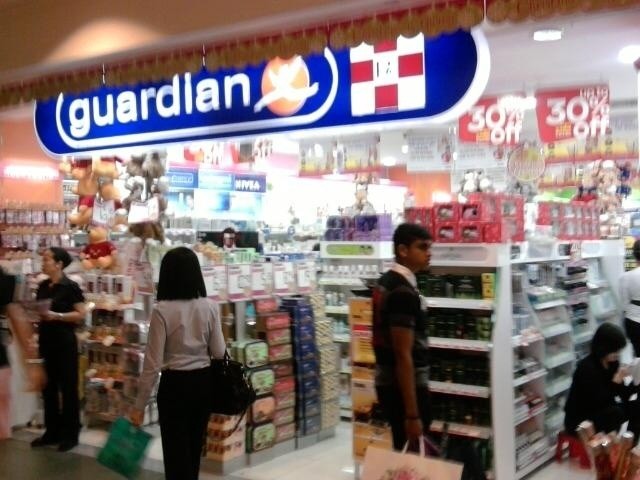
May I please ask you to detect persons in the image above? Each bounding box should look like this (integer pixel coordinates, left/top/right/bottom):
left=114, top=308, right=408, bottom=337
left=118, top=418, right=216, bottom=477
left=562, top=320, right=639, bottom=450
left=371, top=221, right=442, bottom=461
left=132, top=246, right=226, bottom=479
left=23, top=248, right=89, bottom=454
left=616, top=241, right=639, bottom=353
left=222, top=227, right=234, bottom=248
left=0, top=240, right=35, bottom=444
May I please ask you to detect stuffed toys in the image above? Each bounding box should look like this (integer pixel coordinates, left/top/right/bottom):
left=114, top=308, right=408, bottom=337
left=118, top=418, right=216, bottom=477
left=57, top=150, right=169, bottom=271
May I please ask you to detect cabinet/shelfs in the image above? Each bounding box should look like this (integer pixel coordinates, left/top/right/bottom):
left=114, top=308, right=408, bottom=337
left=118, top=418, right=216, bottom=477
left=0, top=243, right=627, bottom=480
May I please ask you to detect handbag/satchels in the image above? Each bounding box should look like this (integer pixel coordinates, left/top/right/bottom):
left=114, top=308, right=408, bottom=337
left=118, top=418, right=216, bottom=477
left=97, top=416, right=152, bottom=479
left=210, top=350, right=257, bottom=415
left=359, top=445, right=465, bottom=480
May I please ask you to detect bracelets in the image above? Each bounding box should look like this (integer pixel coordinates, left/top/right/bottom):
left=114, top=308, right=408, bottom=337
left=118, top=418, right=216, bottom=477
left=57, top=313, right=63, bottom=320
left=403, top=413, right=420, bottom=420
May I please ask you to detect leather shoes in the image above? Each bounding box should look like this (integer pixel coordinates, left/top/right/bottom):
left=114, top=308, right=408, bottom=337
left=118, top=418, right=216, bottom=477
left=30, top=434, right=78, bottom=451
